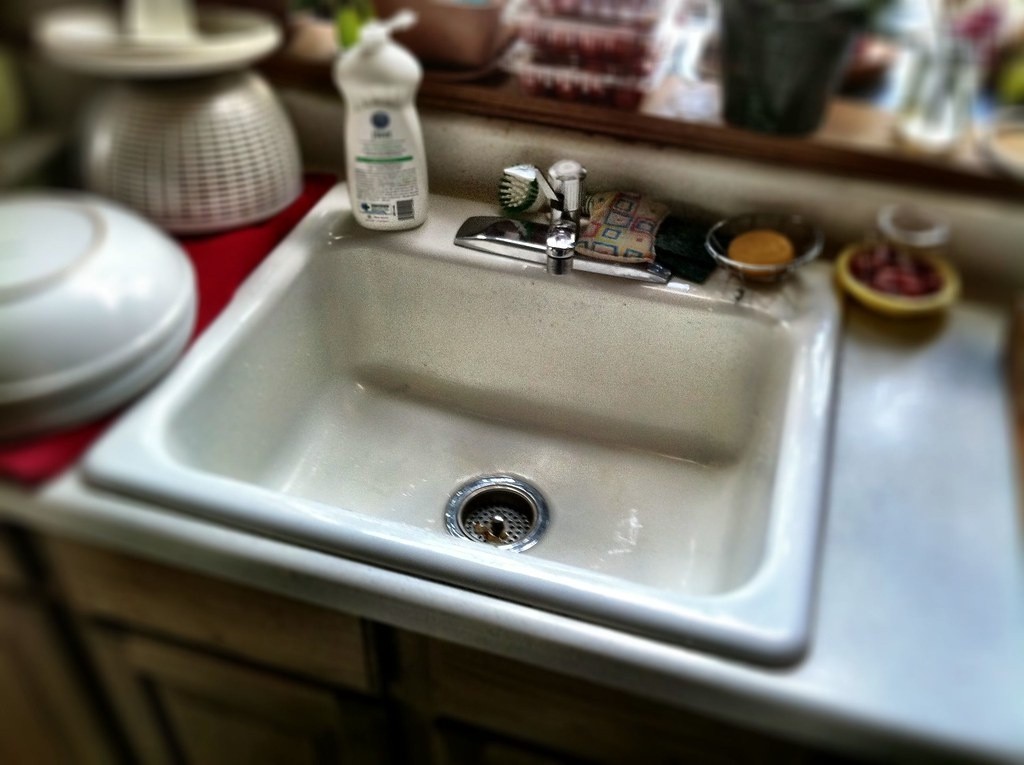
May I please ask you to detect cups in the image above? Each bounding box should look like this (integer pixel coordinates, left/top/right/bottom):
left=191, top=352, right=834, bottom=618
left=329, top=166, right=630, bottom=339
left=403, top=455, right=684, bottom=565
left=713, top=1, right=875, bottom=136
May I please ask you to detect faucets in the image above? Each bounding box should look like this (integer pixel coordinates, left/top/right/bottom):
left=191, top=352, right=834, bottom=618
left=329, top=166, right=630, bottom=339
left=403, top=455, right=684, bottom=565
left=519, top=156, right=587, bottom=282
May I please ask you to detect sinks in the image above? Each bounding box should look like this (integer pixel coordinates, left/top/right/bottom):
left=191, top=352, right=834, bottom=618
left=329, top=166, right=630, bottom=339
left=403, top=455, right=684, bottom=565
left=77, top=173, right=864, bottom=677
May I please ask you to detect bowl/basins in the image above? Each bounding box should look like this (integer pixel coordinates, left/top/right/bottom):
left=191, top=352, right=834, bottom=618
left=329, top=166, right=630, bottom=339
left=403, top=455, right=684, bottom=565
left=2, top=189, right=201, bottom=441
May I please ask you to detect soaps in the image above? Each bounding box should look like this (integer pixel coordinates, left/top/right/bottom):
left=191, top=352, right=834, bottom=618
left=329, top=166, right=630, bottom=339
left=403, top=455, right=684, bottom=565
left=727, top=228, right=801, bottom=271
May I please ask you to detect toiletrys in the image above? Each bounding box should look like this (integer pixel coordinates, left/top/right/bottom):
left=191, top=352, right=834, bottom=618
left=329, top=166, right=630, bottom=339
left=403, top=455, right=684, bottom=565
left=337, top=7, right=430, bottom=238
